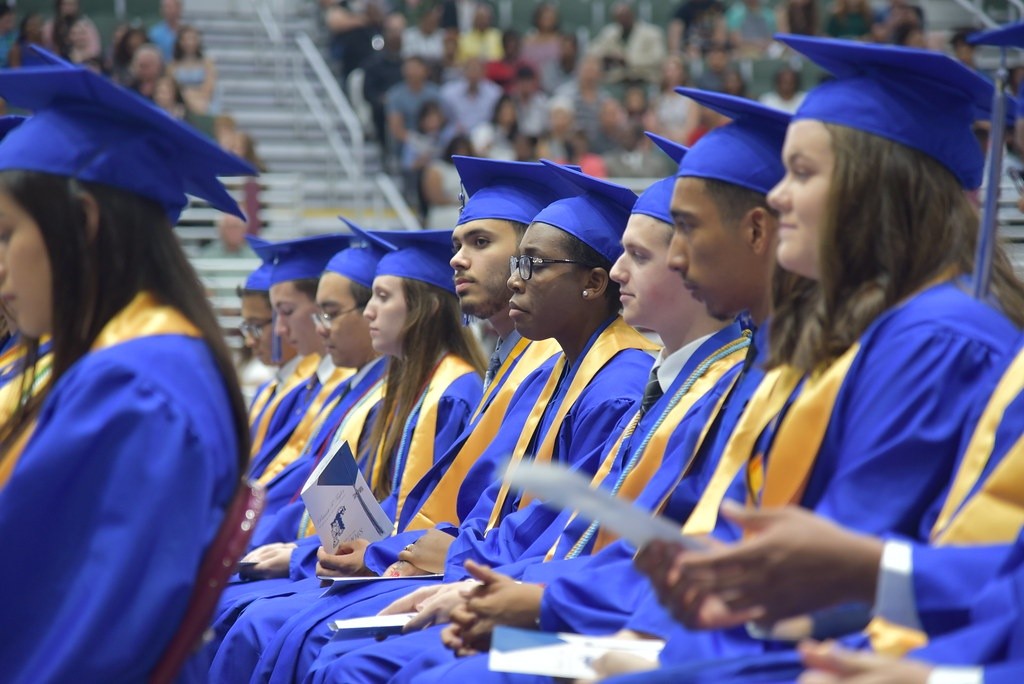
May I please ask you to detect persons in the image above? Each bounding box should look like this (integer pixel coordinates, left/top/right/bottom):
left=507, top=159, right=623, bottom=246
left=0, top=17, right=1024, bottom=684
left=0, top=0, right=1024, bottom=204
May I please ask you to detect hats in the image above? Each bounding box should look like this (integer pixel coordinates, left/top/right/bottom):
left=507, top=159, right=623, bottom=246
left=630, top=131, right=689, bottom=227
left=0, top=43, right=260, bottom=228
left=321, top=215, right=397, bottom=291
left=243, top=234, right=274, bottom=291
left=774, top=32, right=1017, bottom=192
left=452, top=155, right=583, bottom=226
left=966, top=19, right=1024, bottom=121
left=255, top=232, right=355, bottom=285
left=674, top=86, right=795, bottom=196
left=531, top=159, right=639, bottom=266
left=367, top=230, right=456, bottom=295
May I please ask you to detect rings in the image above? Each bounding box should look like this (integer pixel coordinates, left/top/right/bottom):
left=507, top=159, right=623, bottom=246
left=406, top=544, right=414, bottom=551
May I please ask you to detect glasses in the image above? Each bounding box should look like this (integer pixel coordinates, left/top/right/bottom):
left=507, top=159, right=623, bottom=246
left=238, top=319, right=272, bottom=338
left=509, top=256, right=579, bottom=281
left=310, top=306, right=361, bottom=329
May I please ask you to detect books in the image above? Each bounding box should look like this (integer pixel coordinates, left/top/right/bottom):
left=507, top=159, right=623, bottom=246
left=488, top=625, right=665, bottom=680
left=327, top=612, right=419, bottom=642
left=317, top=576, right=444, bottom=599
